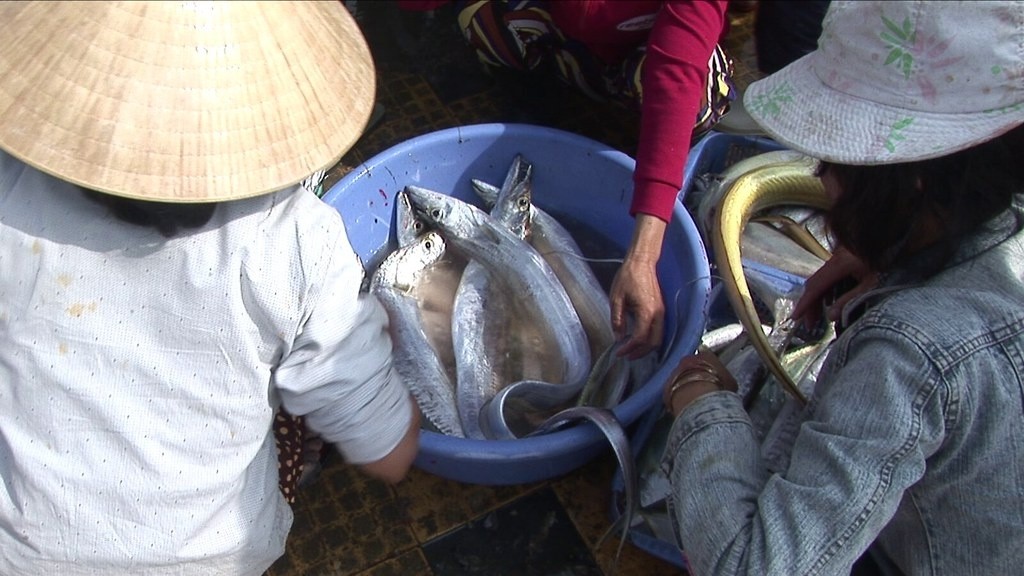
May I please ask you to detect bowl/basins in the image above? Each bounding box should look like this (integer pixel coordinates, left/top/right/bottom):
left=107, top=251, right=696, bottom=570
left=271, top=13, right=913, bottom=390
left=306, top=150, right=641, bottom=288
left=320, top=124, right=712, bottom=486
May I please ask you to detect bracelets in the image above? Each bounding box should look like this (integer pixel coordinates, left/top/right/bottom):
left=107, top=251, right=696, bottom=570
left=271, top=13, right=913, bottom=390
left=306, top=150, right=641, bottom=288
left=670, top=360, right=722, bottom=398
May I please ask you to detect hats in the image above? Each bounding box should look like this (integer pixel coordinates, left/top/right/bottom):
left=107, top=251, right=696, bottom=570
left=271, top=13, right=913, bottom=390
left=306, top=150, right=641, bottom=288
left=742, top=0, right=1024, bottom=167
left=0, top=0, right=378, bottom=203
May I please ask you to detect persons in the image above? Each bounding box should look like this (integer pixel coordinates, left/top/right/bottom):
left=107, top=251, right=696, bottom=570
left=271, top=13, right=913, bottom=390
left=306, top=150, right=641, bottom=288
left=394, top=0, right=738, bottom=360
left=664, top=0, right=1024, bottom=576
left=2, top=1, right=413, bottom=576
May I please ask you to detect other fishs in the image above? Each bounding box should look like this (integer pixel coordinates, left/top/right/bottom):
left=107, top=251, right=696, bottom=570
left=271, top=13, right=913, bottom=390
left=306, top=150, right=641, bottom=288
left=626, top=148, right=857, bottom=506
left=370, top=152, right=658, bottom=565
